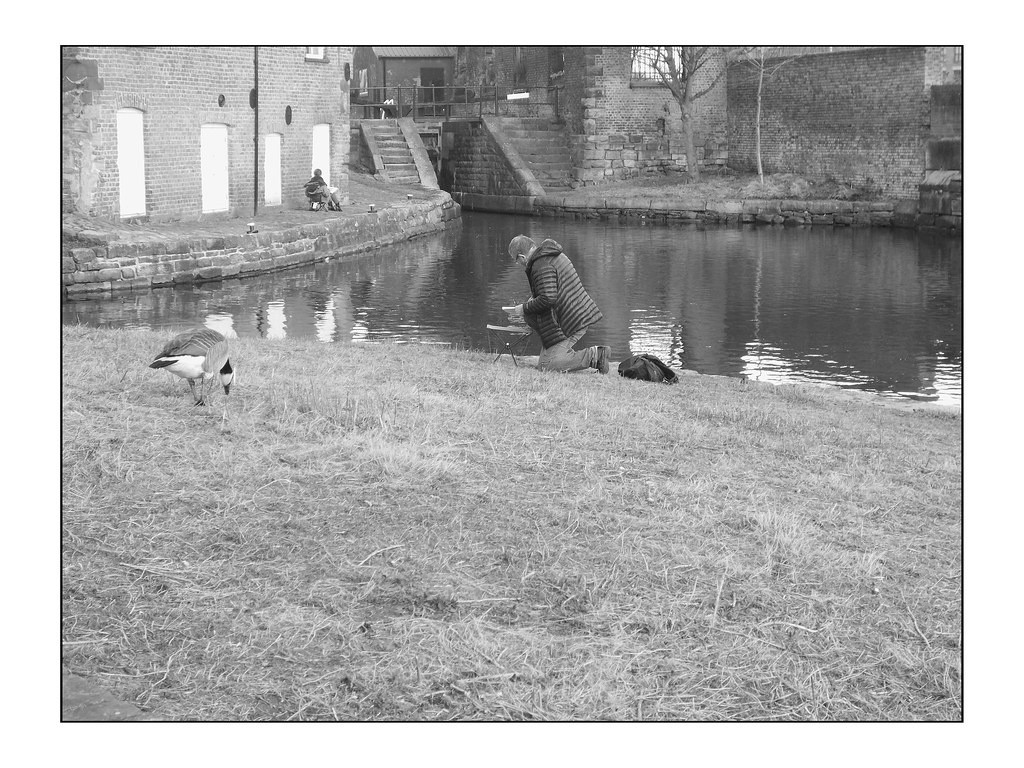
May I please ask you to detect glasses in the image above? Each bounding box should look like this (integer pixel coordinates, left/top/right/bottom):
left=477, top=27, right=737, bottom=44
left=514, top=253, right=519, bottom=265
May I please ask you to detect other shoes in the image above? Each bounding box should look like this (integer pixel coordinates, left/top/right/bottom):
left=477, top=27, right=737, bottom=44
left=593, top=346, right=611, bottom=375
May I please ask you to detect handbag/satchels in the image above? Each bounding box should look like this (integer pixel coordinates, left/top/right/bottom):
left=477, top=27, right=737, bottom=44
left=618, top=353, right=680, bottom=385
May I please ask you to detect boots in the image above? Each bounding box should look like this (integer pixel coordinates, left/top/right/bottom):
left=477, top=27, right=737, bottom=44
left=327, top=201, right=343, bottom=212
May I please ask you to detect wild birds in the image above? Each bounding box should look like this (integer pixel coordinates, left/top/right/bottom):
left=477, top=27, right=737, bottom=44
left=148, top=328, right=234, bottom=407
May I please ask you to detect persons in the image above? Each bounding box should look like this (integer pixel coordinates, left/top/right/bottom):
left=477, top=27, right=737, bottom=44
left=508, top=234, right=612, bottom=375
left=309, top=169, right=342, bottom=211
